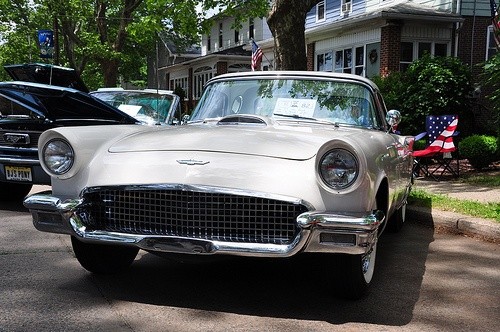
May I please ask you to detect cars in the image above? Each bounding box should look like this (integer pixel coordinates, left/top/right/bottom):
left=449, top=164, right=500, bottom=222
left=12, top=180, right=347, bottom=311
left=23, top=72, right=415, bottom=301
left=0, top=81, right=182, bottom=188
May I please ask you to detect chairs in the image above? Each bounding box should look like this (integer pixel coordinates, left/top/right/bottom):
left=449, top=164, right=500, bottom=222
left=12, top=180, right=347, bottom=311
left=411, top=115, right=460, bottom=181
